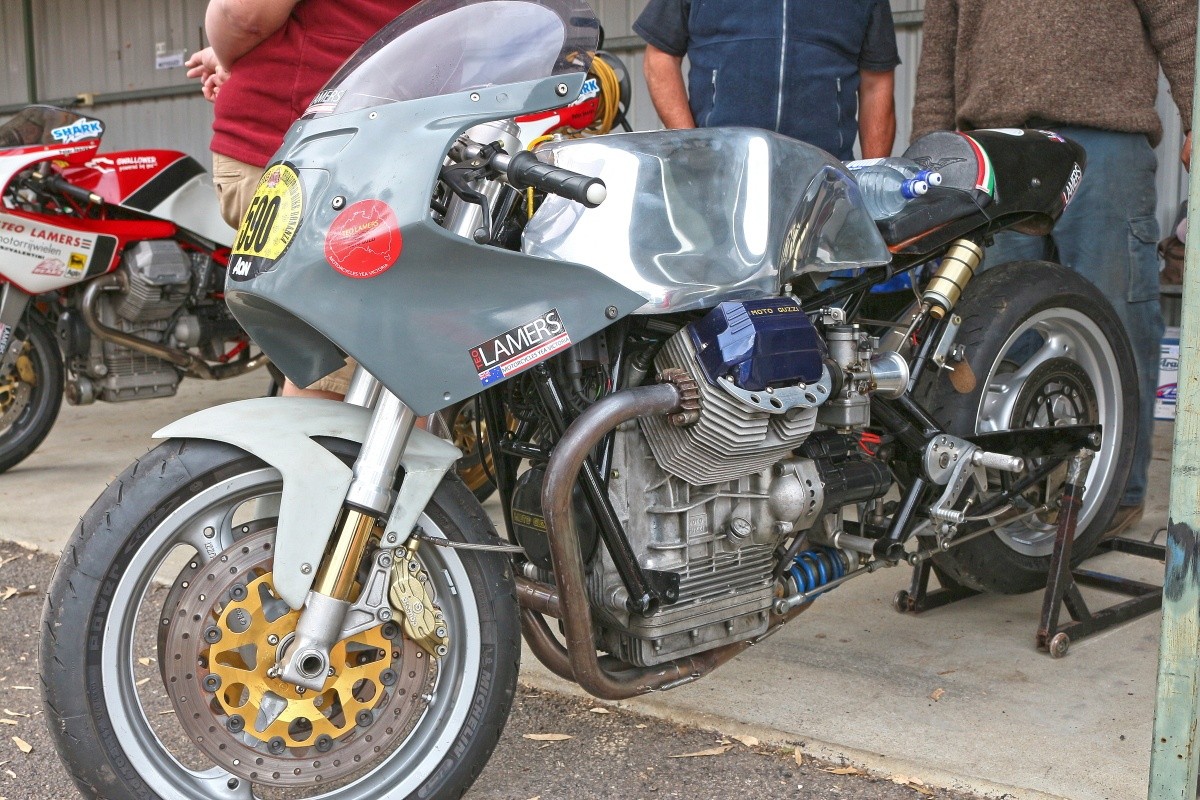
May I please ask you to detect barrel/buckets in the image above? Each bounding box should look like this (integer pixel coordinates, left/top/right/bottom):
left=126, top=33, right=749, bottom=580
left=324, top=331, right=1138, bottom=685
left=1155, top=327, right=1180, bottom=422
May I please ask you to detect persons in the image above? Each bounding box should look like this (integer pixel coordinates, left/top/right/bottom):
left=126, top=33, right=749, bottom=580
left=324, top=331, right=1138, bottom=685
left=907, top=0, right=1200, bottom=547
left=182, top=0, right=472, bottom=730
left=631, top=0, right=905, bottom=297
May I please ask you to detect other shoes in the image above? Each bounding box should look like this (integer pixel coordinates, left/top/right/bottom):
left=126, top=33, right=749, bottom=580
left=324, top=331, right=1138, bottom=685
left=1102, top=501, right=1144, bottom=545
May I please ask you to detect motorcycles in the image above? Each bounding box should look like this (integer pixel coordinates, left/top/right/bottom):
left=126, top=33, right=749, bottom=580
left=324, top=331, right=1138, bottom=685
left=30, top=0, right=1149, bottom=798
left=0, top=104, right=276, bottom=478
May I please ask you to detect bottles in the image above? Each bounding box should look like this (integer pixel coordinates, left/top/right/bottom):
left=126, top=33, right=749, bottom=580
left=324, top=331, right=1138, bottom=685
left=844, top=157, right=943, bottom=191
left=844, top=160, right=927, bottom=219
